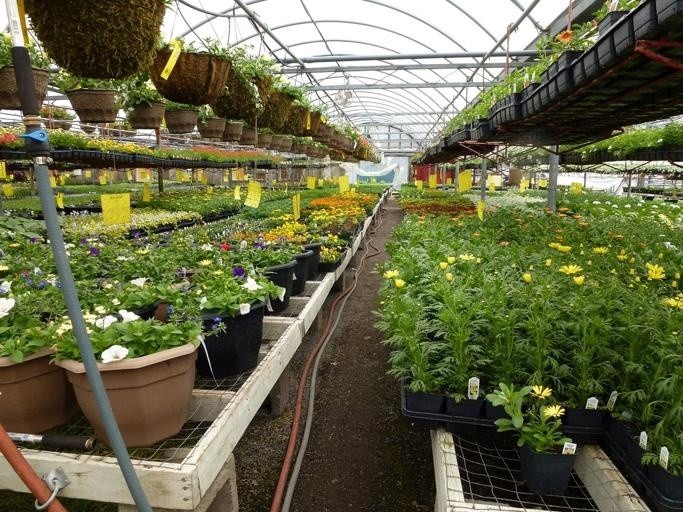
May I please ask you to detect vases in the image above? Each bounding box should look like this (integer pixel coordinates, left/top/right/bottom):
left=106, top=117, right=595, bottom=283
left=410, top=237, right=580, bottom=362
left=613, top=452, right=683, bottom=512
left=0, top=337, right=205, bottom=448
left=522, top=452, right=572, bottom=494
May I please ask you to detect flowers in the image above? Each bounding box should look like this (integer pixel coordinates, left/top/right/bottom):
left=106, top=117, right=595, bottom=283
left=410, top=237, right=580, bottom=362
left=0, top=180, right=392, bottom=362
left=383, top=183, right=683, bottom=452
